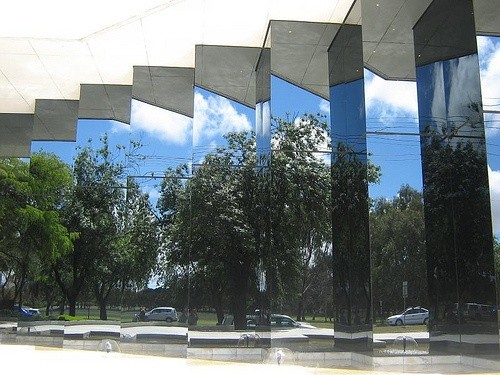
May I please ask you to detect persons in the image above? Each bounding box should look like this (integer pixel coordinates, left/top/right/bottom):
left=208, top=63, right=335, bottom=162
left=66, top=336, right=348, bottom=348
left=276, top=349, right=282, bottom=365
left=137, top=307, right=147, bottom=323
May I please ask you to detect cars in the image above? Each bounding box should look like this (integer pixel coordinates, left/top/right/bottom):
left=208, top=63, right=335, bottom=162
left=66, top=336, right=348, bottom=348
left=387, top=305, right=430, bottom=326
left=145, top=307, right=178, bottom=323
left=247, top=313, right=314, bottom=329
left=447, top=300, right=500, bottom=322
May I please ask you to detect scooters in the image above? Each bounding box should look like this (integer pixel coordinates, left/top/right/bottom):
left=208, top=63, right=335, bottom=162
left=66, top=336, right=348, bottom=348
left=132, top=313, right=150, bottom=322
